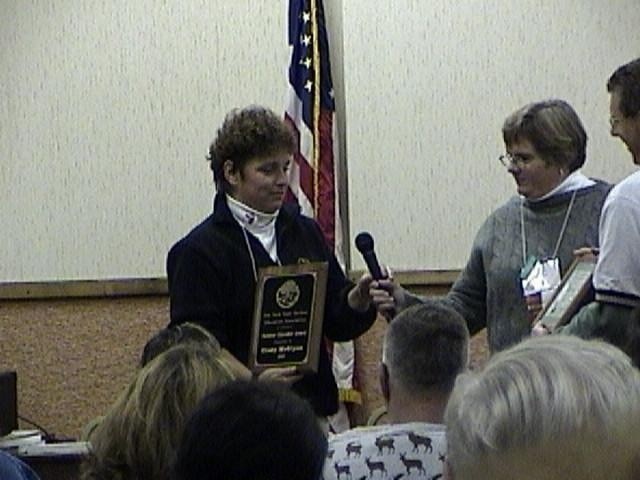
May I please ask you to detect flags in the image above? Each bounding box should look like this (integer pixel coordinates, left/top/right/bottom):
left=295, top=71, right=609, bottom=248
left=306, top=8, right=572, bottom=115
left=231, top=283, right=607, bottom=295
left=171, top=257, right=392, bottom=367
left=285, top=0, right=363, bottom=405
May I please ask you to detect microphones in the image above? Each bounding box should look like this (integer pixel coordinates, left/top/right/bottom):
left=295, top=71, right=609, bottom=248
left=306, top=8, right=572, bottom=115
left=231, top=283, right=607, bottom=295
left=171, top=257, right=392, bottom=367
left=355, top=230, right=383, bottom=282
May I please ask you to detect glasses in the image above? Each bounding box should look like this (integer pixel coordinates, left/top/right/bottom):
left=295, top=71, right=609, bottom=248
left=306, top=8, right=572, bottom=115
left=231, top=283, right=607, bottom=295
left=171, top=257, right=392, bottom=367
left=609, top=116, right=635, bottom=127
left=497, top=152, right=548, bottom=167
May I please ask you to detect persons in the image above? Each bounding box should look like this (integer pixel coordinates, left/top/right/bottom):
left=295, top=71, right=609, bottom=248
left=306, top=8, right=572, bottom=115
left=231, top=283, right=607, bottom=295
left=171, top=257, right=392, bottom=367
left=443, top=334, right=640, bottom=479
left=73, top=341, right=254, bottom=479
left=161, top=102, right=394, bottom=437
left=532, top=56, right=639, bottom=362
left=165, top=376, right=328, bottom=479
left=138, top=320, right=219, bottom=365
left=317, top=303, right=470, bottom=479
left=369, top=99, right=614, bottom=359
